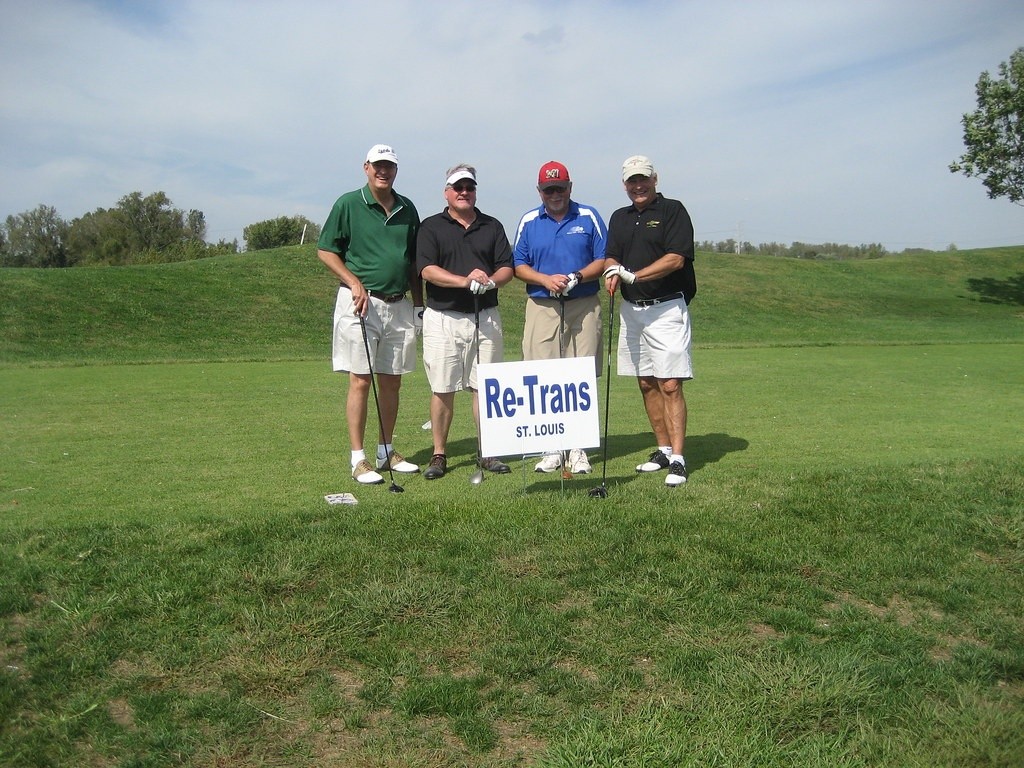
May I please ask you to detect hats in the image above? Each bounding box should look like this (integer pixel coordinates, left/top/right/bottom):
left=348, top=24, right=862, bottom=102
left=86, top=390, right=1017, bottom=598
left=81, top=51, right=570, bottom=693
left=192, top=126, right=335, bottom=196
left=446, top=171, right=477, bottom=186
left=538, top=161, right=570, bottom=189
left=365, top=144, right=398, bottom=165
left=622, top=155, right=655, bottom=182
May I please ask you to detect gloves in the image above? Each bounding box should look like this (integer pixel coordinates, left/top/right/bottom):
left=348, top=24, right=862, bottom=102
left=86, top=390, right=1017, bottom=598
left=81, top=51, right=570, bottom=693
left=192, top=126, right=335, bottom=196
left=413, top=306, right=424, bottom=336
left=602, top=264, right=635, bottom=285
left=470, top=279, right=496, bottom=294
left=549, top=273, right=578, bottom=299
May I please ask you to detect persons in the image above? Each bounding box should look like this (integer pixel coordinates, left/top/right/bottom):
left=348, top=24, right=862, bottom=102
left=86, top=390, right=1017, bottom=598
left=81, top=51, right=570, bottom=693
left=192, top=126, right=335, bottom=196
left=316, top=144, right=420, bottom=483
left=604, top=155, right=698, bottom=487
left=513, top=160, right=607, bottom=474
left=417, top=164, right=514, bottom=480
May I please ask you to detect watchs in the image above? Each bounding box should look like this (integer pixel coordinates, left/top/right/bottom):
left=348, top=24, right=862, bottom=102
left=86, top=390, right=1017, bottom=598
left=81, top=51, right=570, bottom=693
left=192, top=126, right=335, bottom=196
left=572, top=271, right=583, bottom=284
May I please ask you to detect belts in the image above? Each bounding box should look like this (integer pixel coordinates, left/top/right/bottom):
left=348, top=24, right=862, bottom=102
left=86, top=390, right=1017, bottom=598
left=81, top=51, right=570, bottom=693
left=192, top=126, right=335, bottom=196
left=625, top=292, right=685, bottom=307
left=340, top=282, right=405, bottom=303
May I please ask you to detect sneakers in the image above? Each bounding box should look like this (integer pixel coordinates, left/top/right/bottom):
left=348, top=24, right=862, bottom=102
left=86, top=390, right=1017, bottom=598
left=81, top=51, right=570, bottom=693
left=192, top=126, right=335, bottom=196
left=477, top=457, right=511, bottom=473
left=424, top=455, right=446, bottom=479
left=376, top=449, right=420, bottom=473
left=569, top=450, right=592, bottom=474
left=534, top=450, right=566, bottom=472
left=635, top=450, right=671, bottom=472
left=351, top=459, right=384, bottom=484
left=664, top=460, right=687, bottom=486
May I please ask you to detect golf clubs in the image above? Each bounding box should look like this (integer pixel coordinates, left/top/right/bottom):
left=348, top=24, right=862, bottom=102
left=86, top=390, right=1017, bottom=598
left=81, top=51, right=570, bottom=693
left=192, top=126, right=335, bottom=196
left=588, top=288, right=615, bottom=497
left=558, top=294, right=573, bottom=480
left=357, top=308, right=405, bottom=492
left=470, top=291, right=495, bottom=484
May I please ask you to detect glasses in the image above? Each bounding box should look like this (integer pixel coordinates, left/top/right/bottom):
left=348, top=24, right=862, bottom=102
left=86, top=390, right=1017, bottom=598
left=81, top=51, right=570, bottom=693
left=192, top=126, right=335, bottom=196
left=445, top=185, right=476, bottom=192
left=543, top=186, right=567, bottom=195
